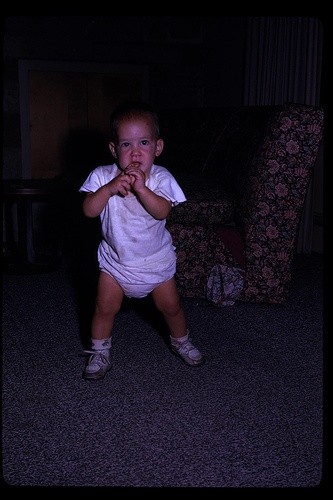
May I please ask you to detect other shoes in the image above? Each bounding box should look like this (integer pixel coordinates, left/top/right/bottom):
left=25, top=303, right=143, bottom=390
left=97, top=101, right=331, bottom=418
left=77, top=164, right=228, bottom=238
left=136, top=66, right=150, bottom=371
left=82, top=349, right=112, bottom=380
left=170, top=341, right=204, bottom=366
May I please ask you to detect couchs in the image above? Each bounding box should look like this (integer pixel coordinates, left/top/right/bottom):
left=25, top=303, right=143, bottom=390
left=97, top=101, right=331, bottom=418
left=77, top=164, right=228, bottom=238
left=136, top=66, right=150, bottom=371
left=134, top=102, right=323, bottom=307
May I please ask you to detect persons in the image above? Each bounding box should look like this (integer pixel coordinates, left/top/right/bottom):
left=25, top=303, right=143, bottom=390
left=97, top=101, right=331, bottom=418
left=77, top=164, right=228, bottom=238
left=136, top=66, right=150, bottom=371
left=79, top=107, right=204, bottom=380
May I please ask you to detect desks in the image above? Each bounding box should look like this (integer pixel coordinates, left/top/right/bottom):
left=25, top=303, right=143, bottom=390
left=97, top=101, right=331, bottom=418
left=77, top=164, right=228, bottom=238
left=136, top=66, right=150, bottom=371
left=2, top=180, right=68, bottom=276
